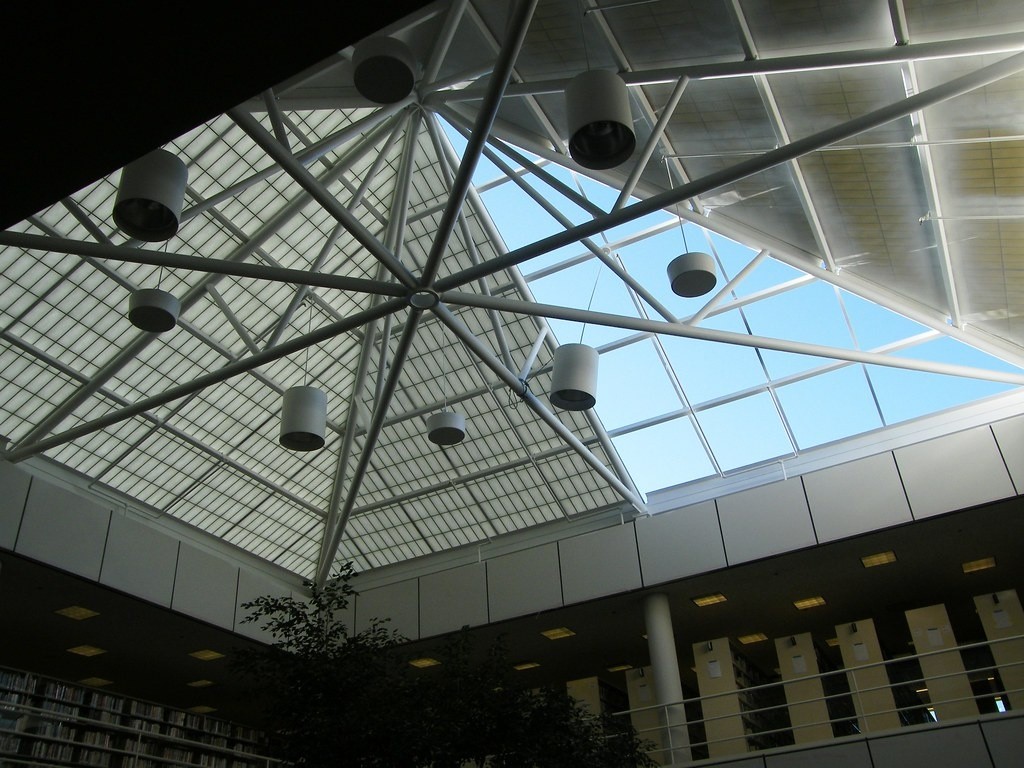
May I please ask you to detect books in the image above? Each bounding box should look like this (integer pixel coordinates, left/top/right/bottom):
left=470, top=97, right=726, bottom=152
left=0, top=670, right=267, bottom=767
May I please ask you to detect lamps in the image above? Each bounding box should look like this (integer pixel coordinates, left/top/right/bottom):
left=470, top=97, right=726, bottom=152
left=129, top=239, right=182, bottom=333
left=549, top=344, right=599, bottom=411
left=113, top=150, right=189, bottom=243
left=426, top=411, right=465, bottom=445
left=564, top=1, right=636, bottom=170
left=279, top=298, right=326, bottom=452
left=666, top=253, right=716, bottom=298
left=351, top=35, right=418, bottom=105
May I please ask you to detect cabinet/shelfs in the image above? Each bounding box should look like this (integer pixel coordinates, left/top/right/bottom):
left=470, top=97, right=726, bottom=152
left=566, top=588, right=1024, bottom=768
left=0, top=665, right=279, bottom=768
